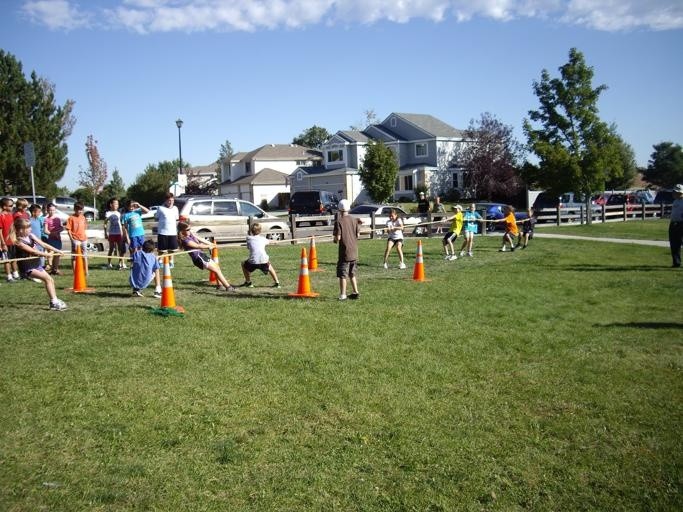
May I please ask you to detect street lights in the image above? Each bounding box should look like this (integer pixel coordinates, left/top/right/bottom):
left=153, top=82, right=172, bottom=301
left=175, top=118, right=182, bottom=197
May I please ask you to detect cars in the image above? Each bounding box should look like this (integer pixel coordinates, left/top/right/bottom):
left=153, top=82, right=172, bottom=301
left=348, top=204, right=429, bottom=237
left=592, top=190, right=680, bottom=220
left=447, top=203, right=528, bottom=232
left=140, top=206, right=161, bottom=219
left=0, top=204, right=109, bottom=252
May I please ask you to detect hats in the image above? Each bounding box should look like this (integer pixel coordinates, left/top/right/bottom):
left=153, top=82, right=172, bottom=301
left=339, top=199, right=350, bottom=210
left=451, top=204, right=463, bottom=211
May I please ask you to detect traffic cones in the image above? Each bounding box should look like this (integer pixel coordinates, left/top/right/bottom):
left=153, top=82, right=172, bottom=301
left=63, top=244, right=94, bottom=293
left=287, top=246, right=320, bottom=298
left=404, top=239, right=431, bottom=282
left=307, top=237, right=322, bottom=272
left=154, top=253, right=184, bottom=315
left=202, top=239, right=230, bottom=286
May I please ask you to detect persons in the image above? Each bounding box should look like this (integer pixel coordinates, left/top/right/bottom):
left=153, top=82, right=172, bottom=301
left=515, top=209, right=535, bottom=250
left=383, top=210, right=406, bottom=270
left=333, top=199, right=359, bottom=300
left=669, top=184, right=683, bottom=268
left=459, top=204, right=482, bottom=257
left=432, top=196, right=447, bottom=234
left=0, top=194, right=179, bottom=311
left=240, top=223, right=280, bottom=289
left=442, top=205, right=464, bottom=261
left=416, top=192, right=431, bottom=233
left=495, top=206, right=518, bottom=251
left=176, top=222, right=233, bottom=292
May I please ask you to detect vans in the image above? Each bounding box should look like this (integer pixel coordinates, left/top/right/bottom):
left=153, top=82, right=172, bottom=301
left=287, top=191, right=339, bottom=227
left=18, top=196, right=100, bottom=222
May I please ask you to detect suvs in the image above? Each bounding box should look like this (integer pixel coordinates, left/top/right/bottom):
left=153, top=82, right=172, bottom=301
left=161, top=194, right=290, bottom=249
left=532, top=192, right=605, bottom=224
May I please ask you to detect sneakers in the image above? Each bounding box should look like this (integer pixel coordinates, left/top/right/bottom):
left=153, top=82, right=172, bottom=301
left=384, top=263, right=388, bottom=269
left=154, top=290, right=163, bottom=298
left=467, top=252, right=473, bottom=257
left=400, top=263, right=405, bottom=269
left=272, top=282, right=281, bottom=289
left=240, top=281, right=253, bottom=288
left=444, top=256, right=457, bottom=261
left=226, top=284, right=238, bottom=291
left=348, top=293, right=359, bottom=300
left=52, top=268, right=64, bottom=275
left=459, top=251, right=465, bottom=257
left=50, top=299, right=67, bottom=310
left=132, top=290, right=143, bottom=297
left=499, top=247, right=505, bottom=252
